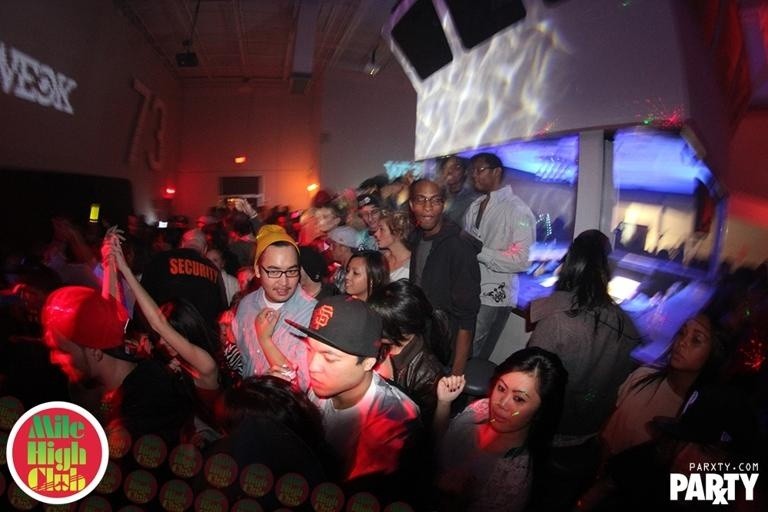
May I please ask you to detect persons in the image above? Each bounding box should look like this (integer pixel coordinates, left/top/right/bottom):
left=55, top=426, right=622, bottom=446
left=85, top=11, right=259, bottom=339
left=0, top=153, right=767, bottom=512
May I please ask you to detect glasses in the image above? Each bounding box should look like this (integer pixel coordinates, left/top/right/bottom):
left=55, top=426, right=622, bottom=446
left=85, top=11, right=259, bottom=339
left=260, top=264, right=301, bottom=278
left=413, top=194, right=443, bottom=206
left=477, top=167, right=492, bottom=173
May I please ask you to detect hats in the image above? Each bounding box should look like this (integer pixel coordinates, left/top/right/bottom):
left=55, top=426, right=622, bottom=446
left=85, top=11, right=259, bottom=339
left=285, top=294, right=384, bottom=356
left=651, top=384, right=759, bottom=458
left=254, top=224, right=300, bottom=266
left=299, top=246, right=326, bottom=282
left=40, top=286, right=144, bottom=364
left=328, top=226, right=360, bottom=248
